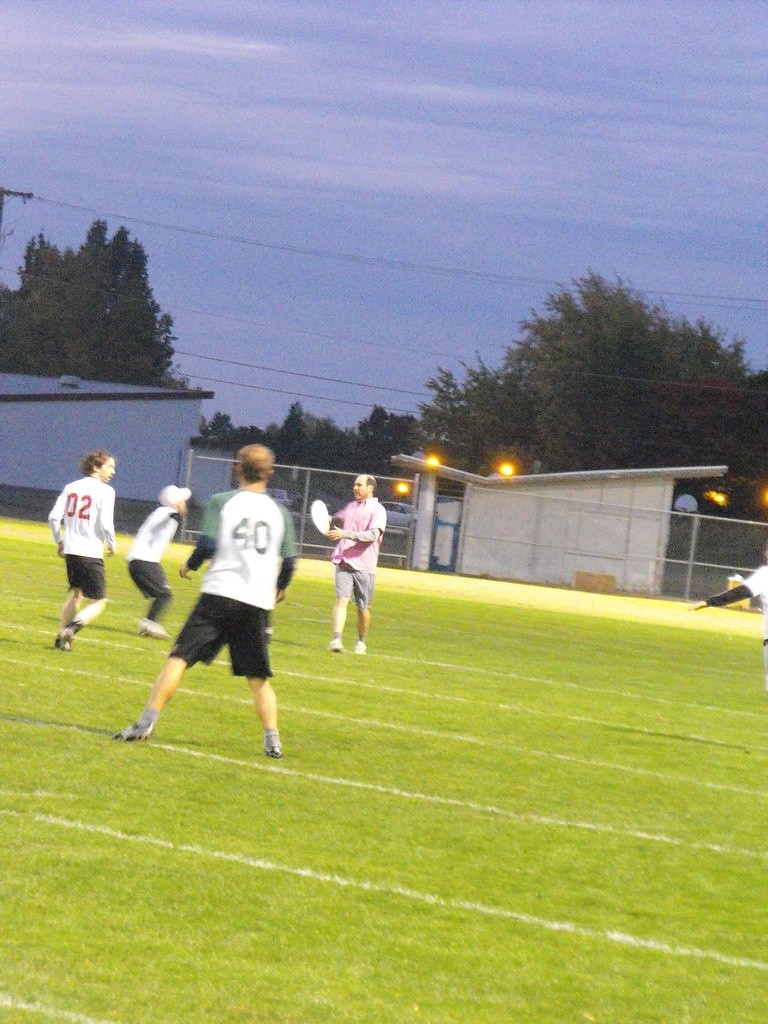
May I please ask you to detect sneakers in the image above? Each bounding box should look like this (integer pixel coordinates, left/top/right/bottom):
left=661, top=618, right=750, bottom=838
left=355, top=643, right=366, bottom=654
left=330, top=639, right=343, bottom=651
left=113, top=726, right=150, bottom=741
left=264, top=746, right=282, bottom=759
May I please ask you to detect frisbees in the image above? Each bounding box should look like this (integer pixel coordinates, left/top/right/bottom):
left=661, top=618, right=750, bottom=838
left=311, top=499, right=331, bottom=536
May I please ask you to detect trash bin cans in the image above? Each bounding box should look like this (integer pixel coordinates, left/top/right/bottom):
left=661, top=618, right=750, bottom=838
left=727, top=577, right=751, bottom=611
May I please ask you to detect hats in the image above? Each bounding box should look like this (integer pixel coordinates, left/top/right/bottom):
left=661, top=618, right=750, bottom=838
left=159, top=485, right=191, bottom=506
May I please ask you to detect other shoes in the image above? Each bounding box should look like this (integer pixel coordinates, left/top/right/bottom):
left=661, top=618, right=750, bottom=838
left=60, top=627, right=73, bottom=652
left=141, top=623, right=169, bottom=639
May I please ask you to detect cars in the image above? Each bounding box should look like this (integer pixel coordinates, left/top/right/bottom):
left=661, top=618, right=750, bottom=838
left=381, top=501, right=413, bottom=538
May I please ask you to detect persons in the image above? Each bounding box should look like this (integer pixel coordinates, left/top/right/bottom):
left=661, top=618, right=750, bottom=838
left=327, top=475, right=387, bottom=654
left=112, top=445, right=295, bottom=759
left=49, top=450, right=116, bottom=651
left=689, top=542, right=768, bottom=690
left=126, top=486, right=191, bottom=637
